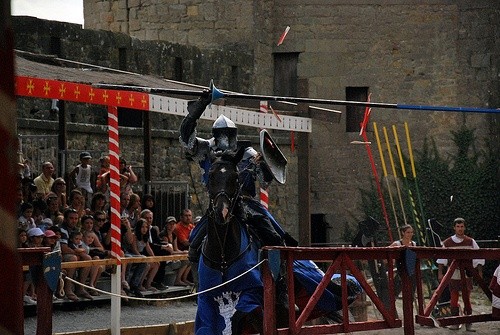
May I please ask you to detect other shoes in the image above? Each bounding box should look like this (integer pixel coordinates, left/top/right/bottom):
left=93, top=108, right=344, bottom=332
left=278, top=292, right=301, bottom=313
left=23, top=289, right=100, bottom=304
left=174, top=280, right=193, bottom=287
left=122, top=280, right=168, bottom=297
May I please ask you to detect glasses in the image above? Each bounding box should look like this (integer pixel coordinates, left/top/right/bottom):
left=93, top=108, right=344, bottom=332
left=57, top=182, right=67, bottom=186
left=96, top=218, right=106, bottom=222
left=67, top=208, right=77, bottom=213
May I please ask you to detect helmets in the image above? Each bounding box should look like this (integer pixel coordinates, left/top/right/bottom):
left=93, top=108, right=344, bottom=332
left=212, top=115, right=237, bottom=150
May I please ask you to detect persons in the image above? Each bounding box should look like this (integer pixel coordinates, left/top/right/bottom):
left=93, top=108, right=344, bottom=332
left=436, top=217, right=484, bottom=332
left=381, top=225, right=417, bottom=299
left=179, top=88, right=287, bottom=292
left=18, top=151, right=201, bottom=304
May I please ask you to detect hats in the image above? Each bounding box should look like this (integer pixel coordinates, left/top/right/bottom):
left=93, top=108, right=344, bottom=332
left=44, top=230, right=61, bottom=239
left=165, top=216, right=177, bottom=224
left=80, top=151, right=92, bottom=160
left=27, top=228, right=45, bottom=238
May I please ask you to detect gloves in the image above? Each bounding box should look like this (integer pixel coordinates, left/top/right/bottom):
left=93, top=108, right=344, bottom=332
left=197, top=88, right=212, bottom=106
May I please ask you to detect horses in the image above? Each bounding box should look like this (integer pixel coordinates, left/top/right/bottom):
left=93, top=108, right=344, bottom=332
left=194, top=147, right=354, bottom=335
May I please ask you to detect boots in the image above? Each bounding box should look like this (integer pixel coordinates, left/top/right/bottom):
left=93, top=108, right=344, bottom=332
left=464, top=307, right=477, bottom=332
left=448, top=307, right=461, bottom=330
